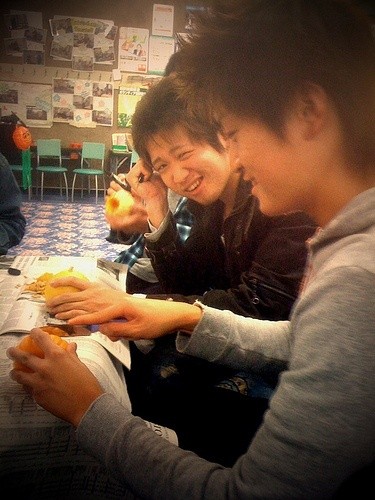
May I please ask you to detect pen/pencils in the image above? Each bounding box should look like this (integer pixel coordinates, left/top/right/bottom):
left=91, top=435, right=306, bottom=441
left=103, top=168, right=132, bottom=194
left=139, top=173, right=145, bottom=183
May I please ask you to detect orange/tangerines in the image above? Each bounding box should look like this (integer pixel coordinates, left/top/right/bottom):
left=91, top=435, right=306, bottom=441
left=45, top=266, right=92, bottom=311
left=14, top=333, right=72, bottom=375
left=103, top=190, right=138, bottom=218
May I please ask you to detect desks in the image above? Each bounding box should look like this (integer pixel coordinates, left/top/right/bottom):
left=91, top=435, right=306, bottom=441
left=0, top=255, right=179, bottom=472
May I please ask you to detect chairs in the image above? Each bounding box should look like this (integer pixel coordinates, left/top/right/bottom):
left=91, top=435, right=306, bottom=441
left=35, top=138, right=106, bottom=204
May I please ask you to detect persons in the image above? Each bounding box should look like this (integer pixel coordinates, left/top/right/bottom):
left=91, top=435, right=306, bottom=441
left=0, top=0, right=375, bottom=500
left=0, top=152, right=26, bottom=256
left=104, top=47, right=318, bottom=441
